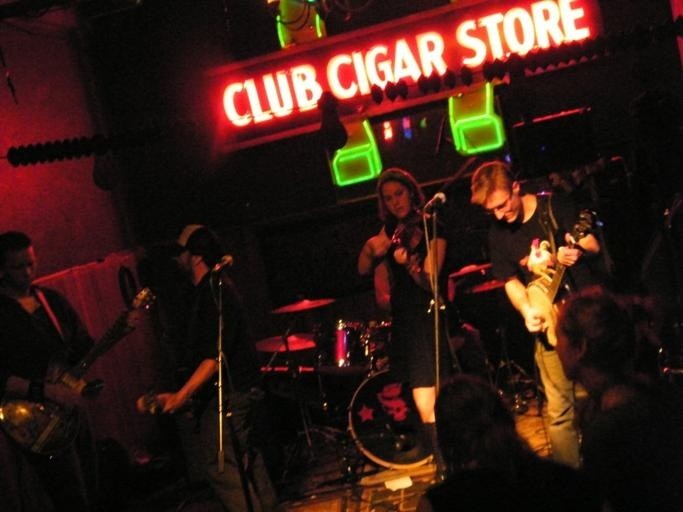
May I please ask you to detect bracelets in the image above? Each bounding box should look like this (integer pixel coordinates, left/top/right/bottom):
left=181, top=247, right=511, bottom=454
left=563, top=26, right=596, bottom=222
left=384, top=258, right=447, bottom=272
left=28, top=379, right=44, bottom=401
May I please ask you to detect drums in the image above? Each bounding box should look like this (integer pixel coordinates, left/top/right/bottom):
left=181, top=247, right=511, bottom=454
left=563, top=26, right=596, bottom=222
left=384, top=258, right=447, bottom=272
left=335, top=319, right=392, bottom=368
left=348, top=368, right=433, bottom=470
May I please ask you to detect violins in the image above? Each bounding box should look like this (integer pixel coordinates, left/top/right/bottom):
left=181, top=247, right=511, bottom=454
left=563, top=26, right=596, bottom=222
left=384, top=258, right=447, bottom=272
left=391, top=206, right=432, bottom=276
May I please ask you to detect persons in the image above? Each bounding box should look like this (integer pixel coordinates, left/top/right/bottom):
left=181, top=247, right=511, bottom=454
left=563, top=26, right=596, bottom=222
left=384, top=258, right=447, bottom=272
left=357, top=167, right=449, bottom=424
left=554, top=291, right=682, bottom=512
left=467, top=159, right=611, bottom=439
left=131, top=221, right=283, bottom=512
left=0, top=228, right=145, bottom=509
left=547, top=135, right=638, bottom=266
left=412, top=378, right=614, bottom=512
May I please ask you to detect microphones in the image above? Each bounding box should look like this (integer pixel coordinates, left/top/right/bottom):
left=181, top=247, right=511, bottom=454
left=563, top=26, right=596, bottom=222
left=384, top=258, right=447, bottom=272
left=423, top=192, right=447, bottom=211
left=213, top=254, right=233, bottom=273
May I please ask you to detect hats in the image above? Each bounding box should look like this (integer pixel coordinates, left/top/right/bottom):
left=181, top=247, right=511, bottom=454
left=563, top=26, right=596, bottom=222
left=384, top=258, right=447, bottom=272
left=175, top=224, right=212, bottom=251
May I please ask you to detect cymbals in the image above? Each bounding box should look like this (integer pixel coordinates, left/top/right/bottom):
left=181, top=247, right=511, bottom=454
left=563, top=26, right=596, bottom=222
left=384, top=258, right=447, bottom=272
left=256, top=332, right=318, bottom=353
left=270, top=298, right=335, bottom=314
left=468, top=279, right=505, bottom=291
left=448, top=263, right=492, bottom=280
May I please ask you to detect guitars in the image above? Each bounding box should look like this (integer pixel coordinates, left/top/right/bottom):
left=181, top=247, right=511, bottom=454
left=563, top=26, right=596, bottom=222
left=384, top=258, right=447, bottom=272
left=0, top=286, right=157, bottom=457
